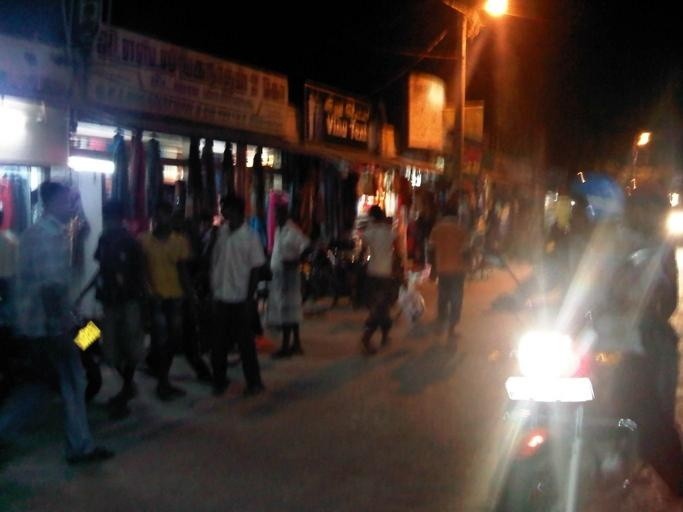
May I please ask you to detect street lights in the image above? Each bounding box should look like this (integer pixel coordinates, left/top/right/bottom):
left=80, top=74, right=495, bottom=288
left=630, top=130, right=652, bottom=191
left=444, top=0, right=510, bottom=188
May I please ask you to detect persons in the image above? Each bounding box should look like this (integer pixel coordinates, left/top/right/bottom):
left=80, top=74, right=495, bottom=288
left=353, top=203, right=403, bottom=356
left=0, top=177, right=314, bottom=470
left=485, top=176, right=683, bottom=503
left=425, top=204, right=471, bottom=340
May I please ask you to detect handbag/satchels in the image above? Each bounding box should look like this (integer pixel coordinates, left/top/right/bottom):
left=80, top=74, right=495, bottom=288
left=398, top=280, right=426, bottom=324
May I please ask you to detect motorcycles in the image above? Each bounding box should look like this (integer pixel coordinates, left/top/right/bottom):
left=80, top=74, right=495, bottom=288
left=481, top=245, right=660, bottom=512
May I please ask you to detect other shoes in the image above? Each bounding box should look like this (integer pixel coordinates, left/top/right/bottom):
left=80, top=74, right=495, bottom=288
left=362, top=335, right=378, bottom=355
left=69, top=328, right=304, bottom=421
left=70, top=445, right=110, bottom=463
left=383, top=336, right=395, bottom=344
